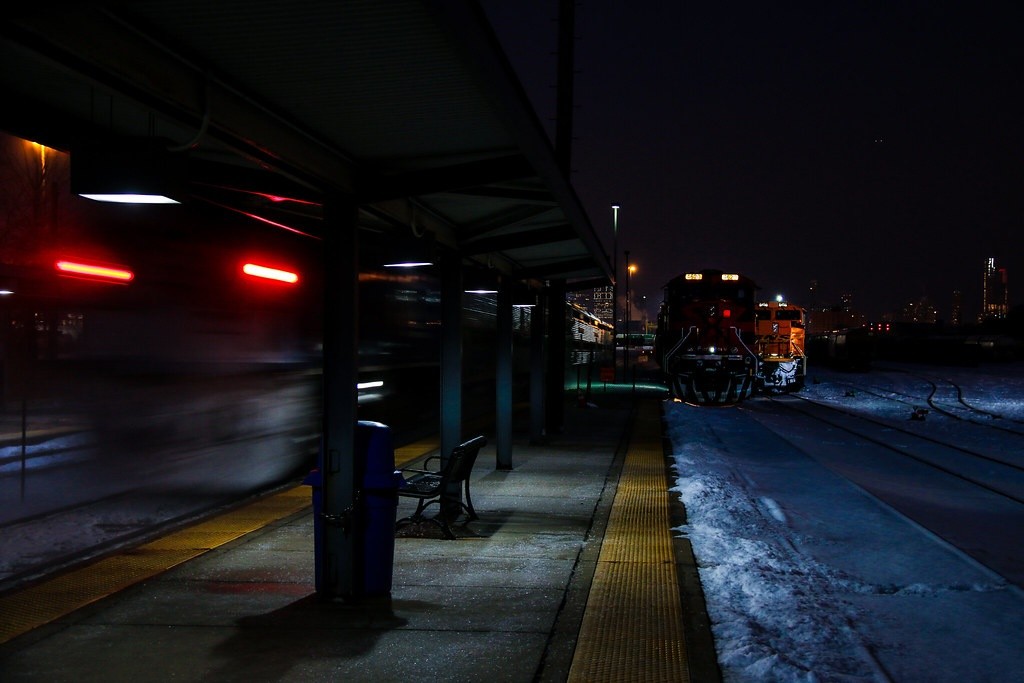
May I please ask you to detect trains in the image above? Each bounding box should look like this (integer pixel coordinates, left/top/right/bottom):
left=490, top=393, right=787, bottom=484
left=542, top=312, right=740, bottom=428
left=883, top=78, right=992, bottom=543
left=570, top=301, right=616, bottom=365
left=654, top=271, right=755, bottom=406
left=758, top=301, right=806, bottom=391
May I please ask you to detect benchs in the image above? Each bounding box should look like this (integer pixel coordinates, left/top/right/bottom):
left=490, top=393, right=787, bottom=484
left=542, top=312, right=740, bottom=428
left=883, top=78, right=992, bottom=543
left=394, top=434, right=486, bottom=541
